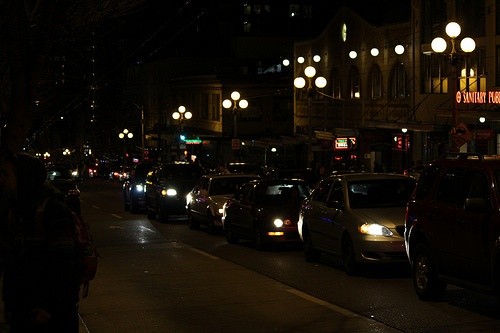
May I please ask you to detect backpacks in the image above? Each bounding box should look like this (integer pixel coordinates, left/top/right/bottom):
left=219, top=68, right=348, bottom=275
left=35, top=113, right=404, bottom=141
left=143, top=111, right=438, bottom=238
left=32, top=197, right=99, bottom=297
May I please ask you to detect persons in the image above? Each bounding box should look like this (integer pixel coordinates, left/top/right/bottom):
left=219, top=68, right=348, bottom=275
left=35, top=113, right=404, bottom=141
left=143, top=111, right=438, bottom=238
left=0, top=150, right=79, bottom=333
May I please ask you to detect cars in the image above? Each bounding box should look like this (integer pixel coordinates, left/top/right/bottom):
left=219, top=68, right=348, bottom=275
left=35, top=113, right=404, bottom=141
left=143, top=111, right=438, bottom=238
left=224, top=178, right=317, bottom=250
left=187, top=173, right=260, bottom=230
left=302, top=172, right=419, bottom=277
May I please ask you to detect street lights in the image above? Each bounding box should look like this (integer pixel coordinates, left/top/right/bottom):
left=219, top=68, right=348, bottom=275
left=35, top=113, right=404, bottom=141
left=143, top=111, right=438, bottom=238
left=221, top=90, right=249, bottom=163
left=118, top=128, right=134, bottom=172
left=172, top=105, right=193, bottom=161
left=430, top=21, right=477, bottom=154
left=293, top=65, right=328, bottom=170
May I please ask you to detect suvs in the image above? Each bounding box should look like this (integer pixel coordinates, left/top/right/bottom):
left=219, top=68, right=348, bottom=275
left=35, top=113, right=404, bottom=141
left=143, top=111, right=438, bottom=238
left=403, top=151, right=500, bottom=302
left=45, top=163, right=79, bottom=187
left=121, top=163, right=156, bottom=215
left=143, top=163, right=206, bottom=223
